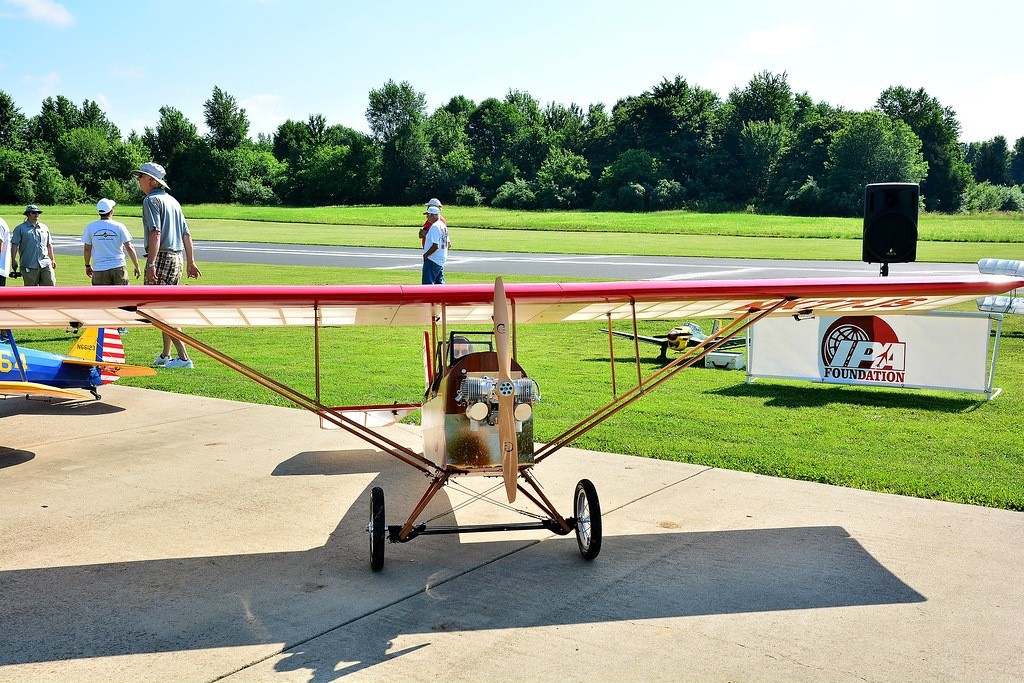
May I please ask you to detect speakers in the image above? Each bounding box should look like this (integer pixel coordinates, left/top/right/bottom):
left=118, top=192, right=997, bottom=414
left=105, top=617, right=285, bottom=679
left=861, top=182, right=920, bottom=265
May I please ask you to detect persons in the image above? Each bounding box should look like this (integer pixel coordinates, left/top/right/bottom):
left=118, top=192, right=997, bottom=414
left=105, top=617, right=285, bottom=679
left=10, top=206, right=56, bottom=286
left=81, top=199, right=141, bottom=334
left=132, top=162, right=202, bottom=368
left=419, top=199, right=451, bottom=284
left=0, top=217, right=11, bottom=343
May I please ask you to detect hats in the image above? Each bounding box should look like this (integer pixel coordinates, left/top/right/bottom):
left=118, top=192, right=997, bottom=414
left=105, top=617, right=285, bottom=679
left=131, top=162, right=171, bottom=192
left=23, top=205, right=42, bottom=214
left=424, top=198, right=443, bottom=207
left=423, top=206, right=440, bottom=215
left=97, top=197, right=116, bottom=214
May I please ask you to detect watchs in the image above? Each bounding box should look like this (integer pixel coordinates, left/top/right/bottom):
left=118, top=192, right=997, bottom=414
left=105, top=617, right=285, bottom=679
left=147, top=263, right=156, bottom=268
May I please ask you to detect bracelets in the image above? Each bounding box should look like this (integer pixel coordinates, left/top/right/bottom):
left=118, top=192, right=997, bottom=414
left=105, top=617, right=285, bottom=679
left=85, top=264, right=91, bottom=267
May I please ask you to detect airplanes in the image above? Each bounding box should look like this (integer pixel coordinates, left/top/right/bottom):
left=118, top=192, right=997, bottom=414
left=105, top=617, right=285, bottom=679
left=598, top=320, right=752, bottom=361
left=0, top=324, right=160, bottom=402
left=0, top=263, right=1024, bottom=573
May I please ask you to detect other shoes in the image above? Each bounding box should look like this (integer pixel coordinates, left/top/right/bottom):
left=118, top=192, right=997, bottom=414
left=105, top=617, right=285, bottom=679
left=118, top=327, right=127, bottom=332
left=66, top=327, right=78, bottom=334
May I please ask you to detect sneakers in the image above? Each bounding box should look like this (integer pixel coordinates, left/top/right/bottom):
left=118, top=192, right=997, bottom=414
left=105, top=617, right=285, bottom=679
left=150, top=356, right=172, bottom=367
left=166, top=356, right=193, bottom=369
left=0, top=334, right=9, bottom=343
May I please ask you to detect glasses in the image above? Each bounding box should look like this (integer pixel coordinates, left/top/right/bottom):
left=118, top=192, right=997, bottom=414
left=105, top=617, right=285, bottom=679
left=139, top=173, right=150, bottom=179
left=30, top=211, right=41, bottom=214
